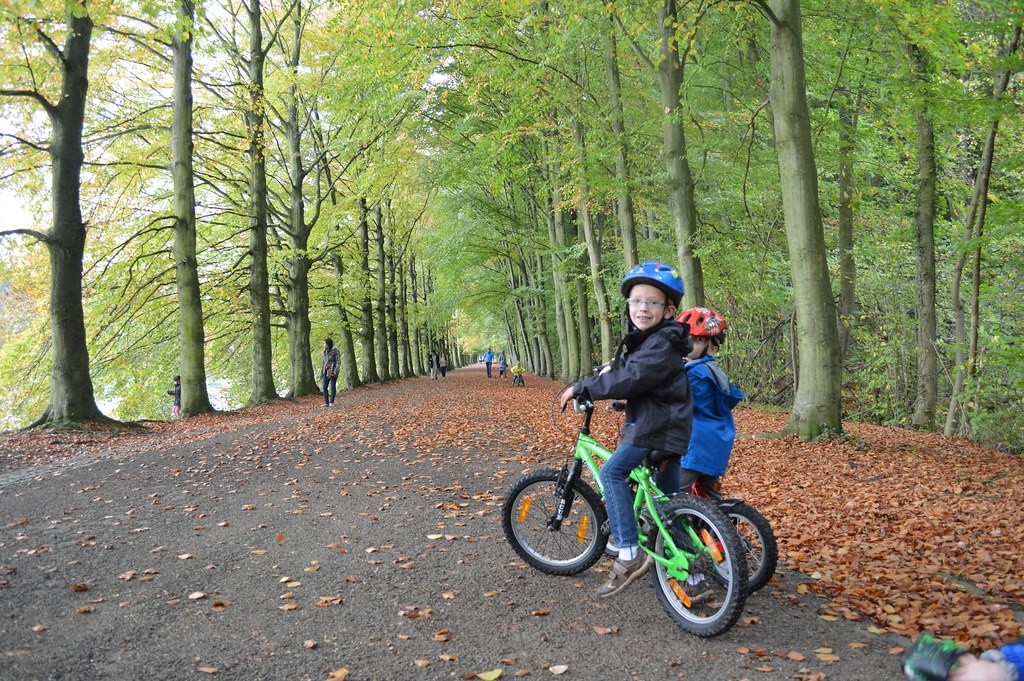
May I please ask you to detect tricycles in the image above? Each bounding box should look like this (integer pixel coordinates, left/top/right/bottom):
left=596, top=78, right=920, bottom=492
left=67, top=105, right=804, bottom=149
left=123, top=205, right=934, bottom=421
left=510, top=370, right=525, bottom=388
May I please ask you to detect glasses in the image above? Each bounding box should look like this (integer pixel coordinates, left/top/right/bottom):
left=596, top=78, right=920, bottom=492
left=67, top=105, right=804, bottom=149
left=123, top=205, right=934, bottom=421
left=626, top=296, right=668, bottom=308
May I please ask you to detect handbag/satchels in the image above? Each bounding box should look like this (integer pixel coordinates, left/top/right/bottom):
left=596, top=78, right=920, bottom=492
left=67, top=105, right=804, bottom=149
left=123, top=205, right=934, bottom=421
left=327, top=369, right=337, bottom=379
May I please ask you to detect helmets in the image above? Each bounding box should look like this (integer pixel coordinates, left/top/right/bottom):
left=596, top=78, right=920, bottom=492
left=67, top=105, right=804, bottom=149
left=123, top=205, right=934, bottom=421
left=621, top=261, right=684, bottom=306
left=675, top=307, right=728, bottom=344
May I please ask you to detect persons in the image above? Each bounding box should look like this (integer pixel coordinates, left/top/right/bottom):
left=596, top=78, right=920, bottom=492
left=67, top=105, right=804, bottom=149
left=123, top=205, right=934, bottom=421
left=499, top=351, right=508, bottom=378
left=428, top=350, right=439, bottom=380
left=499, top=360, right=505, bottom=378
left=675, top=306, right=747, bottom=502
left=320, top=338, right=341, bottom=407
left=558, top=261, right=695, bottom=598
left=438, top=352, right=446, bottom=377
left=483, top=348, right=494, bottom=378
left=948, top=635, right=1024, bottom=681
left=166, top=375, right=181, bottom=418
left=510, top=361, right=526, bottom=387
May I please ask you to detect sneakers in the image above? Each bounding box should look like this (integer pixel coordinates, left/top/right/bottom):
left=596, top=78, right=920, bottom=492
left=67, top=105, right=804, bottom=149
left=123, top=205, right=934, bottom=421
left=596, top=545, right=654, bottom=598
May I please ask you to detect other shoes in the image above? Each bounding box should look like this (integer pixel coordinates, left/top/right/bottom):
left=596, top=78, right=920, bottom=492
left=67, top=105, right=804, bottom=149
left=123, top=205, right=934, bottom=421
left=321, top=402, right=329, bottom=407
left=329, top=403, right=333, bottom=407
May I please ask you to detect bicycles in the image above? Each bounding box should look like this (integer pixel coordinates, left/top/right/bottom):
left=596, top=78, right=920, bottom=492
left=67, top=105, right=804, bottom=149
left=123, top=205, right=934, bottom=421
left=499, top=356, right=780, bottom=639
